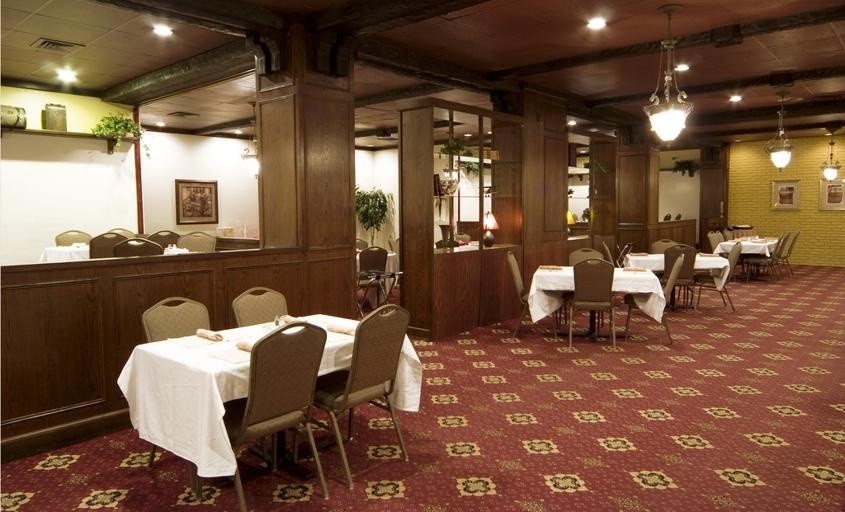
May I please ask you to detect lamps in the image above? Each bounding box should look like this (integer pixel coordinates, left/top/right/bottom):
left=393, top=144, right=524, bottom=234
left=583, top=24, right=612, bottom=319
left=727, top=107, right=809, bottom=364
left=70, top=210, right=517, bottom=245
left=641, top=3, right=694, bottom=146
left=482, top=211, right=500, bottom=247
left=567, top=210, right=576, bottom=237
left=819, top=126, right=844, bottom=181
left=764, top=91, right=794, bottom=171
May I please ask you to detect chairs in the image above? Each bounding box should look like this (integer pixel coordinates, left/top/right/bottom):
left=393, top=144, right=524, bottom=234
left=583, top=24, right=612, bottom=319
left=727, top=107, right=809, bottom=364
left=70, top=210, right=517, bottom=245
left=292, top=304, right=414, bottom=491
left=139, top=294, right=214, bottom=471
left=355, top=235, right=403, bottom=311
left=231, top=286, right=290, bottom=326
left=504, top=222, right=802, bottom=347
left=183, top=319, right=330, bottom=512
left=39, top=227, right=220, bottom=259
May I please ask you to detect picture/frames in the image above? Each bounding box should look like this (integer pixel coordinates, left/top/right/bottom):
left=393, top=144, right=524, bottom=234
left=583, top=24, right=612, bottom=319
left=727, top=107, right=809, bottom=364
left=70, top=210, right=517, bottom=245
left=819, top=178, right=845, bottom=212
left=770, top=179, right=802, bottom=212
left=174, top=177, right=219, bottom=226
